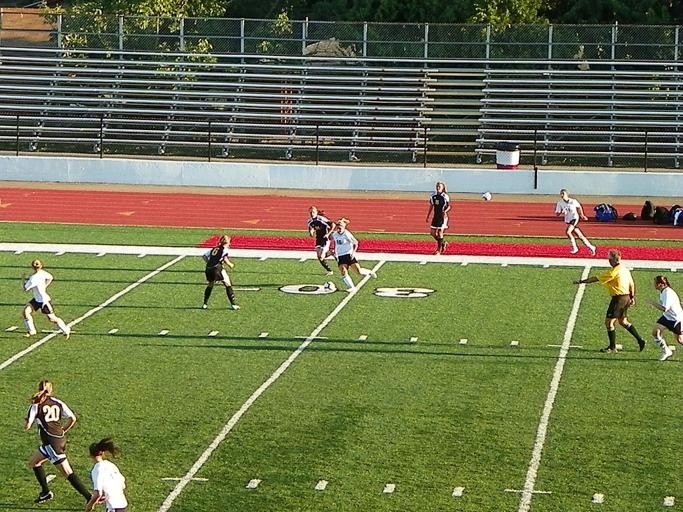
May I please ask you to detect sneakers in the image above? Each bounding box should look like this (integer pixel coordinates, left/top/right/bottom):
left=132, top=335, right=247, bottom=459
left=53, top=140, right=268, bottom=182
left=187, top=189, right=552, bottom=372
left=600, top=346, right=617, bottom=353
left=639, top=340, right=645, bottom=351
left=660, top=351, right=673, bottom=360
left=34, top=492, right=53, bottom=503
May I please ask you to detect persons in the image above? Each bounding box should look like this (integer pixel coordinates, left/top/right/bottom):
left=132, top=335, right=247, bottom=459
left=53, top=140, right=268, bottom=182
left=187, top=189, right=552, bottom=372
left=85, top=436, right=128, bottom=512
left=426, top=182, right=449, bottom=257
left=570, top=248, right=646, bottom=354
left=553, top=186, right=597, bottom=256
left=19, top=258, right=70, bottom=341
left=646, top=274, right=682, bottom=360
left=307, top=205, right=338, bottom=275
left=199, top=233, right=240, bottom=310
left=327, top=215, right=376, bottom=293
left=21, top=379, right=91, bottom=503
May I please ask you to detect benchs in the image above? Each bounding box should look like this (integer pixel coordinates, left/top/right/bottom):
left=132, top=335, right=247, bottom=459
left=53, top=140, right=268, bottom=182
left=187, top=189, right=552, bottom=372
left=0, top=39, right=681, bottom=176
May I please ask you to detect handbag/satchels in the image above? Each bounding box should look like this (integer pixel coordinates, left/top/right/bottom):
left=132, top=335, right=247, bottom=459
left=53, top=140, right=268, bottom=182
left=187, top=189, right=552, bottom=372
left=593, top=201, right=682, bottom=226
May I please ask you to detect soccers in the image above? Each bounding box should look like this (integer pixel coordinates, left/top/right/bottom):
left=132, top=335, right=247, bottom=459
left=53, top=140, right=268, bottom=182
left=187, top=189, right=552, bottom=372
left=482, top=192, right=492, bottom=202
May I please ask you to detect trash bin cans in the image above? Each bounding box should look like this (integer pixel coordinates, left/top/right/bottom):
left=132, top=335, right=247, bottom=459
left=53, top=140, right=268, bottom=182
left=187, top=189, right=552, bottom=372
left=496, top=142, right=521, bottom=170
